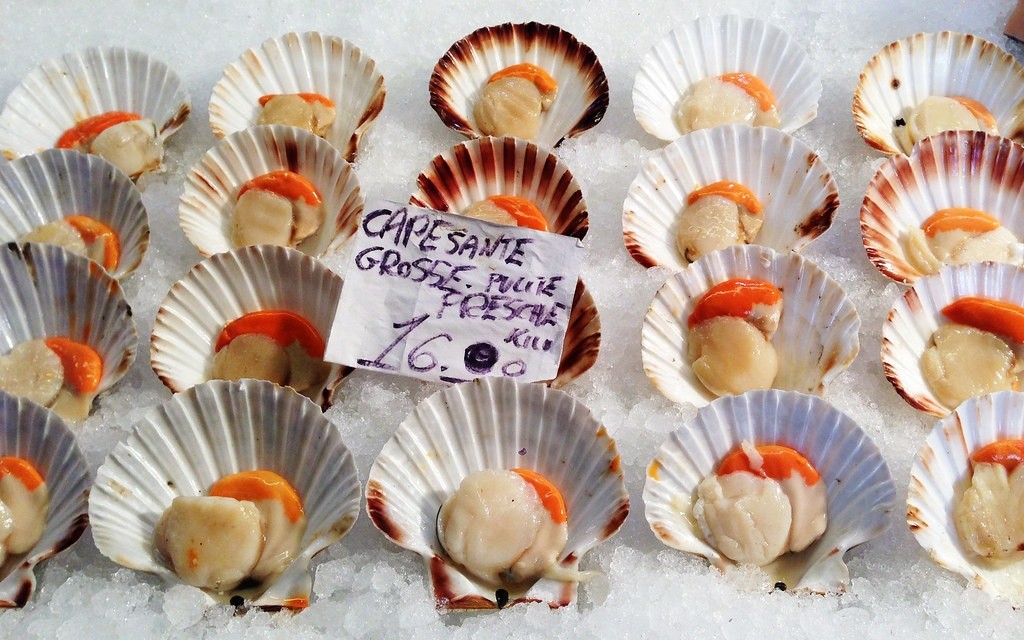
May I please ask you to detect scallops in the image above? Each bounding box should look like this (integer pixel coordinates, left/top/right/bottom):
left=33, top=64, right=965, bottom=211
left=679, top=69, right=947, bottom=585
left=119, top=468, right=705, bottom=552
left=0, top=15, right=1024, bottom=612
